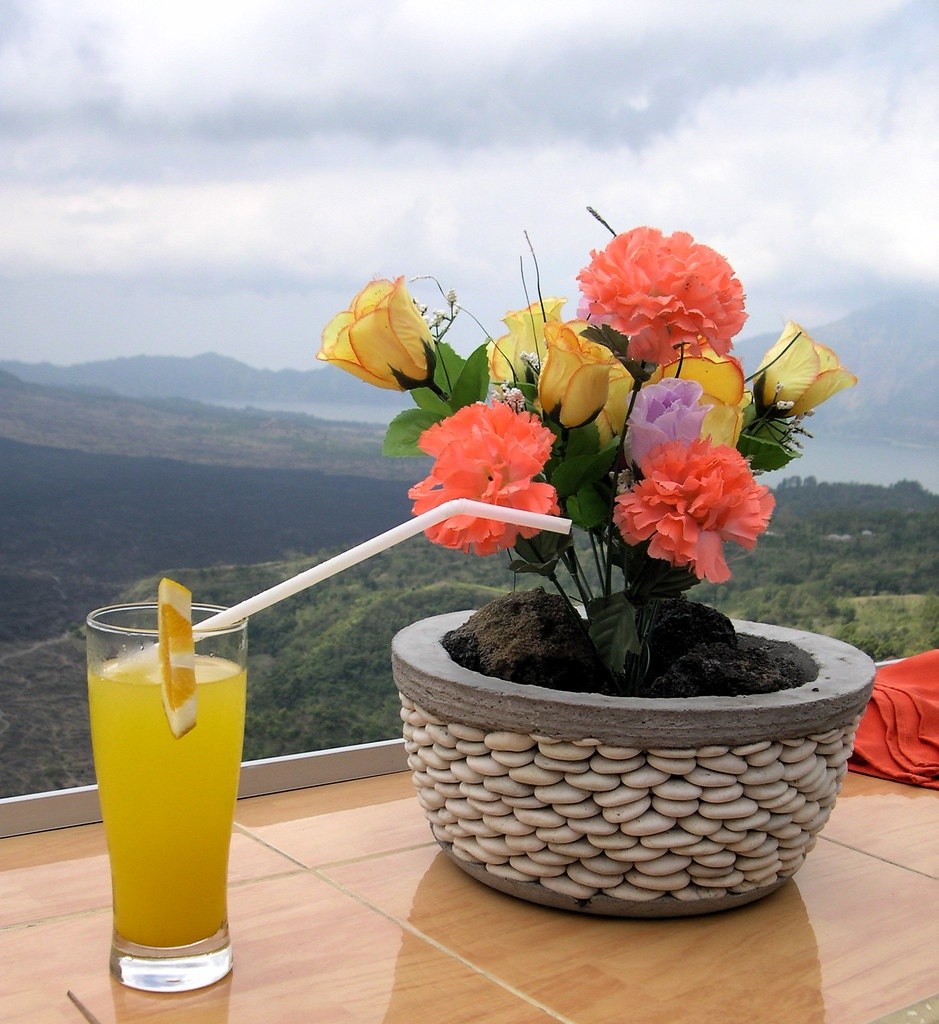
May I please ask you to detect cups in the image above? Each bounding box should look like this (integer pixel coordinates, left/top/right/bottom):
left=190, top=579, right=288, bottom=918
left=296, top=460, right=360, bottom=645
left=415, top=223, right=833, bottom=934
left=85, top=601, right=249, bottom=994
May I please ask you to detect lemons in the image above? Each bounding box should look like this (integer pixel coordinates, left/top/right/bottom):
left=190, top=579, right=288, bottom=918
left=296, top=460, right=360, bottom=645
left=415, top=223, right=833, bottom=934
left=157, top=576, right=197, bottom=740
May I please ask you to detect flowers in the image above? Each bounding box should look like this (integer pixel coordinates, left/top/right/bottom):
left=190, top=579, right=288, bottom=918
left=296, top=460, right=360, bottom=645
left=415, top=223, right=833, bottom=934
left=315, top=206, right=857, bottom=676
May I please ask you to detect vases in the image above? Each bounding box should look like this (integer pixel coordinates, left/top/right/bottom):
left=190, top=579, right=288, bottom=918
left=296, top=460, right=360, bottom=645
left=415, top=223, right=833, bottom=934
left=389, top=610, right=875, bottom=921
